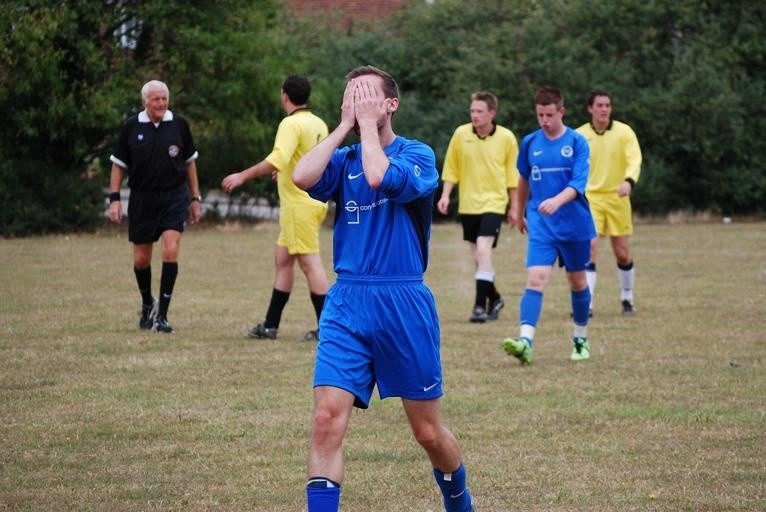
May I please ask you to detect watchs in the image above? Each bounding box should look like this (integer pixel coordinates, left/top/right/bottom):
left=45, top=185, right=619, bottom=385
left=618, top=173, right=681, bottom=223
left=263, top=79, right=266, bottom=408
left=192, top=195, right=202, bottom=203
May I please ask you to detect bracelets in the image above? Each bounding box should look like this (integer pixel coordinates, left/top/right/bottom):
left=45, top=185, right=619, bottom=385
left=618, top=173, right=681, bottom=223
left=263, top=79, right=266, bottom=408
left=110, top=192, right=120, bottom=204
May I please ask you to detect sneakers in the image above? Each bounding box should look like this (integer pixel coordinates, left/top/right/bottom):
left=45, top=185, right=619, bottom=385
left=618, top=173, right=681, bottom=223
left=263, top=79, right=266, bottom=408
left=470, top=295, right=503, bottom=322
left=623, top=301, right=636, bottom=316
left=247, top=325, right=278, bottom=339
left=503, top=338, right=532, bottom=364
left=139, top=298, right=172, bottom=332
left=571, top=340, right=589, bottom=360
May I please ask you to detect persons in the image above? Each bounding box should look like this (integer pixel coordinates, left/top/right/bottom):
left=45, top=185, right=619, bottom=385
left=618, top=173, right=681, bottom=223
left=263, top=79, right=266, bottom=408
left=570, top=87, right=643, bottom=318
left=501, top=86, right=597, bottom=365
left=291, top=65, right=477, bottom=512
left=109, top=80, right=201, bottom=333
left=221, top=75, right=330, bottom=342
left=437, top=93, right=520, bottom=321
